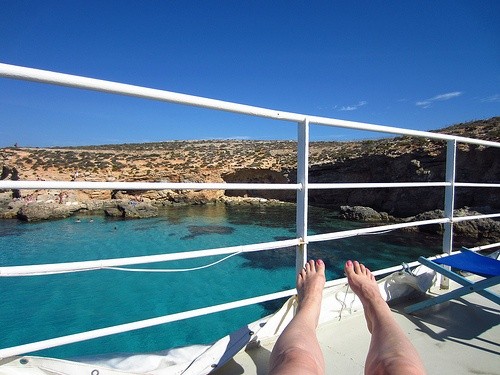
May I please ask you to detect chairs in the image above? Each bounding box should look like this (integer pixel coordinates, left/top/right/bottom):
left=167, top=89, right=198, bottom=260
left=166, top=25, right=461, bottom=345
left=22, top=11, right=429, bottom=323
left=403, top=246, right=500, bottom=314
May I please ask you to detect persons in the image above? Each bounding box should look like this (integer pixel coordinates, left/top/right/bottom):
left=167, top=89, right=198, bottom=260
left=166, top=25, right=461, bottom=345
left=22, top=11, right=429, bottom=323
left=69, top=167, right=79, bottom=181
left=264, top=258, right=425, bottom=375
left=74, top=217, right=117, bottom=232
left=12, top=189, right=70, bottom=204
left=128, top=192, right=145, bottom=203
